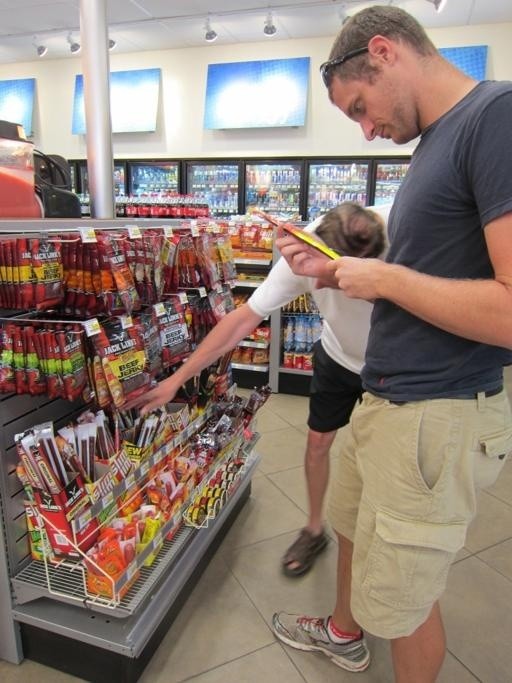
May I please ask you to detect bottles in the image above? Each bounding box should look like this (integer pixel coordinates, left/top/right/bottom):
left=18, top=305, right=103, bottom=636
left=77, top=160, right=407, bottom=218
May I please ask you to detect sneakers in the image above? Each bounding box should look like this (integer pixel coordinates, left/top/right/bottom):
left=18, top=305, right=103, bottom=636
left=272, top=611, right=370, bottom=673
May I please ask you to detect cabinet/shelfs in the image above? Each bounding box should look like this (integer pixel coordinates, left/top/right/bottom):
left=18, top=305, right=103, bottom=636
left=215, top=219, right=323, bottom=394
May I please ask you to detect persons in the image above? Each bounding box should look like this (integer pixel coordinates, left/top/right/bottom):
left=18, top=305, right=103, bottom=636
left=270, top=5, right=510, bottom=683
left=116, top=197, right=393, bottom=581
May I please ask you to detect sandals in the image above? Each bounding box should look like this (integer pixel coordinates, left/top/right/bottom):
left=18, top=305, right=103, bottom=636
left=284, top=526, right=327, bottom=576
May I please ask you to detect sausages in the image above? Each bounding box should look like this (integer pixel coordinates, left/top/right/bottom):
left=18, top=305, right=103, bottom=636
left=85, top=480, right=172, bottom=577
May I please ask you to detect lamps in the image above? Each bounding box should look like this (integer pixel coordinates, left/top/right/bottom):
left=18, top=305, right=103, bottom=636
left=0, top=0, right=445, bottom=58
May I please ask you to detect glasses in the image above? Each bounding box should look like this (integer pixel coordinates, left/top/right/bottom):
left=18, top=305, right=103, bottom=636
left=319, top=47, right=369, bottom=87
